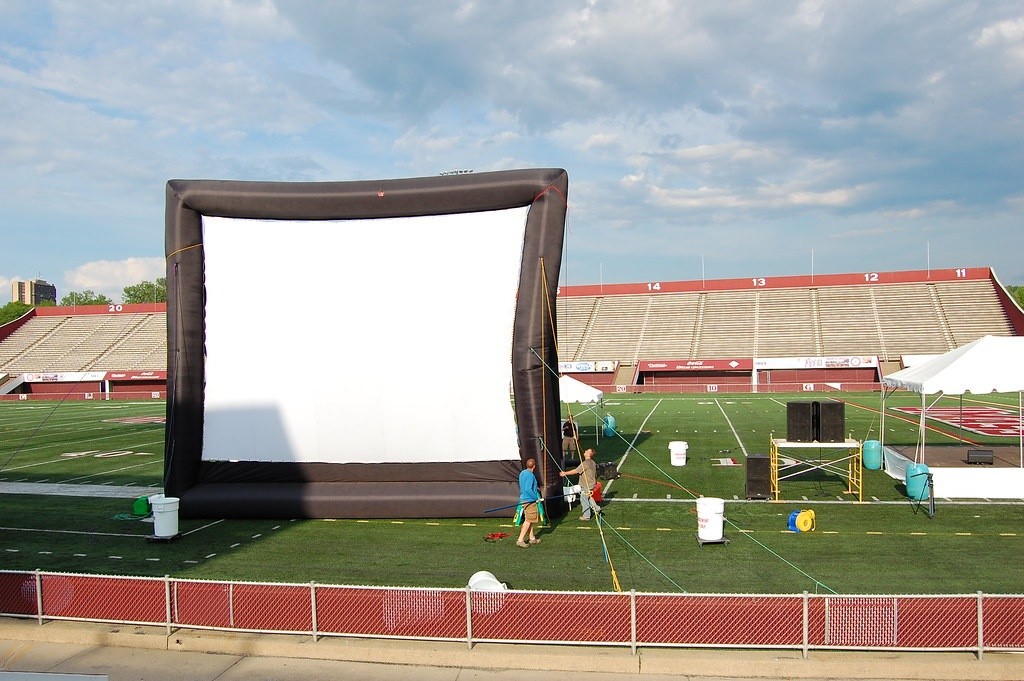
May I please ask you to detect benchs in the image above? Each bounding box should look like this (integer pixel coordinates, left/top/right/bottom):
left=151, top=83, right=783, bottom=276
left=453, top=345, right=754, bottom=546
left=0, top=279, right=1017, bottom=377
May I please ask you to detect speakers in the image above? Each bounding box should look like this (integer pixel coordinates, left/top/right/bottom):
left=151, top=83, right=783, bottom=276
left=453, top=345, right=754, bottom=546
left=967, top=449, right=994, bottom=465
left=785, top=399, right=845, bottom=443
left=746, top=452, right=771, bottom=498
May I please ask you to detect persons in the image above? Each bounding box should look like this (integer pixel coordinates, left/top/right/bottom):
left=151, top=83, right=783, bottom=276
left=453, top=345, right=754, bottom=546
left=559, top=449, right=601, bottom=521
left=562, top=415, right=577, bottom=461
left=516, top=459, right=543, bottom=547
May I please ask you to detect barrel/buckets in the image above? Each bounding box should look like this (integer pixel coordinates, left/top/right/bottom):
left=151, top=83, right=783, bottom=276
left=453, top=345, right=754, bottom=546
left=467, top=571, right=508, bottom=614
left=696, top=497, right=725, bottom=540
left=863, top=440, right=881, bottom=470
left=668, top=440, right=689, bottom=466
left=151, top=497, right=180, bottom=536
left=906, top=463, right=929, bottom=501
left=603, top=415, right=616, bottom=436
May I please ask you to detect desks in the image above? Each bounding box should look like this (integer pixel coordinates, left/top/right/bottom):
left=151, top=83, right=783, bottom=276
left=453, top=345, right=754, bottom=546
left=769, top=432, right=863, bottom=501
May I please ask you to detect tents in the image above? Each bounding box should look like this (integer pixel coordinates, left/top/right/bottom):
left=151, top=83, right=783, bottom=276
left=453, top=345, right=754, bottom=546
left=559, top=375, right=604, bottom=445
left=881, top=335, right=1024, bottom=471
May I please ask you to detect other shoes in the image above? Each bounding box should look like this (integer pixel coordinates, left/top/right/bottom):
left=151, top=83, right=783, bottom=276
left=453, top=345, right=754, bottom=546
left=579, top=515, right=590, bottom=520
left=529, top=539, right=542, bottom=544
left=516, top=540, right=529, bottom=548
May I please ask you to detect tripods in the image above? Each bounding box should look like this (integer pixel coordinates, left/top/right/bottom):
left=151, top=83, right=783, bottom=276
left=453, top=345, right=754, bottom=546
left=911, top=473, right=935, bottom=519
